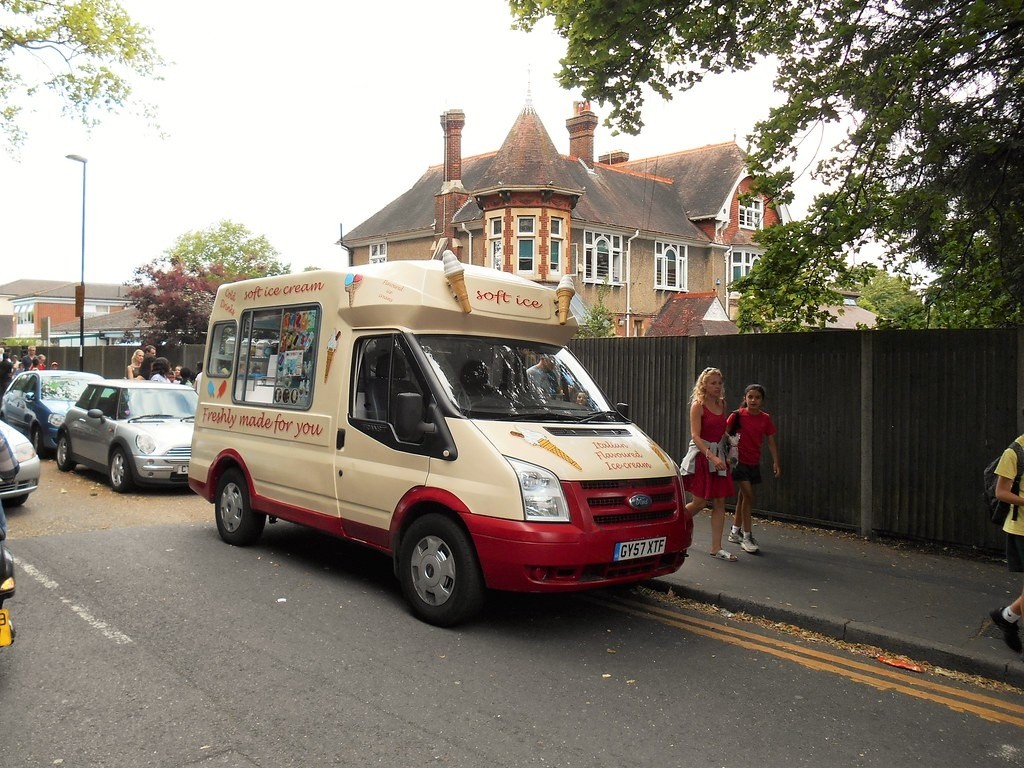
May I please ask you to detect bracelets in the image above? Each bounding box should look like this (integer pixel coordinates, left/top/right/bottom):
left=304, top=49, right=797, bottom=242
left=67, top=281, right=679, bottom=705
left=706, top=448, right=709, bottom=459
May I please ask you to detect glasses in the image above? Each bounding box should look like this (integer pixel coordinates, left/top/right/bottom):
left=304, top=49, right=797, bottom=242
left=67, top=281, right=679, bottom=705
left=701, top=368, right=713, bottom=382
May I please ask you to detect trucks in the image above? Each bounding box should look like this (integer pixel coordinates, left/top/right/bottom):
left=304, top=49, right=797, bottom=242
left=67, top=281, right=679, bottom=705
left=186, top=249, right=695, bottom=633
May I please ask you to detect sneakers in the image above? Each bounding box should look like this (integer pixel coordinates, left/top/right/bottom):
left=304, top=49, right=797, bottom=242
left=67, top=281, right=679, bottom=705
left=740, top=538, right=760, bottom=554
left=728, top=530, right=744, bottom=543
left=990, top=606, right=1022, bottom=654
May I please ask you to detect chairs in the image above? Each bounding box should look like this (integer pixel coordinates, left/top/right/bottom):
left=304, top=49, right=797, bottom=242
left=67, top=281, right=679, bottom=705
left=365, top=352, right=421, bottom=423
left=459, top=361, right=508, bottom=407
left=104, top=391, right=131, bottom=421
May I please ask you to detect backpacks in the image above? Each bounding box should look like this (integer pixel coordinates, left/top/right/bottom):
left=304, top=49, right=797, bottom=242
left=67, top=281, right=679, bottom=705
left=984, top=441, right=1024, bottom=528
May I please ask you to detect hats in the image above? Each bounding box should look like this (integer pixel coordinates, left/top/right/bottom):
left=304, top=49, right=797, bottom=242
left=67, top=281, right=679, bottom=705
left=11, top=355, right=17, bottom=359
left=52, top=362, right=58, bottom=366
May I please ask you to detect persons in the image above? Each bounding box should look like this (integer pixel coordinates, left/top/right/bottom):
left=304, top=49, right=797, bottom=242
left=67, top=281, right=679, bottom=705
left=0, top=431, right=19, bottom=542
left=128, top=349, right=144, bottom=379
left=0, top=345, right=46, bottom=399
left=51, top=362, right=58, bottom=369
left=140, top=345, right=203, bottom=394
left=726, top=384, right=781, bottom=553
left=522, top=348, right=589, bottom=407
left=679, top=367, right=739, bottom=561
left=988, top=408, right=1024, bottom=651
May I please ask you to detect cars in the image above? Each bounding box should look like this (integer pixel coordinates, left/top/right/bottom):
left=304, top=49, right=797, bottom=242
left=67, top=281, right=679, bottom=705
left=55, top=378, right=199, bottom=493
left=0, top=370, right=106, bottom=460
left=0, top=419, right=41, bottom=506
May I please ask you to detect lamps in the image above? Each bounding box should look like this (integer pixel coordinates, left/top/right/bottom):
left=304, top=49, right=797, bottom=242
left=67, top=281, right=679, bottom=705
left=716, top=279, right=721, bottom=286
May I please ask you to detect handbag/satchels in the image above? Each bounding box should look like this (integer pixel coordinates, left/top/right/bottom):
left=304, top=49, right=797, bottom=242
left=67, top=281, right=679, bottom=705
left=725, top=410, right=741, bottom=454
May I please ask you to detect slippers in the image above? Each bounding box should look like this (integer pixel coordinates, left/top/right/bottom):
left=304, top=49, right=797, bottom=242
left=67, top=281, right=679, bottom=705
left=711, top=549, right=737, bottom=562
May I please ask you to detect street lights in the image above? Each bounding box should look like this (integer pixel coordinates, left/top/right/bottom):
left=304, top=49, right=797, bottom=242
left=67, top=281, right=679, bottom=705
left=65, top=154, right=88, bottom=373
